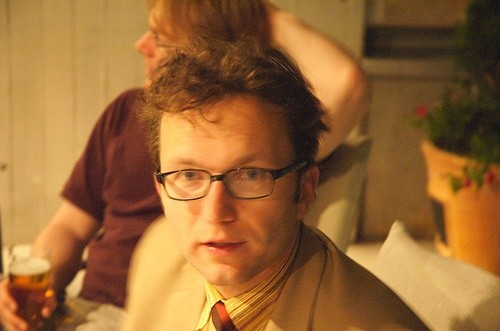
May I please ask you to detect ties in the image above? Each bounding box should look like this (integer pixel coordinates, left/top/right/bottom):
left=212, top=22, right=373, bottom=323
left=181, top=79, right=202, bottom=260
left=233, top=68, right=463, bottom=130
left=209, top=302, right=236, bottom=331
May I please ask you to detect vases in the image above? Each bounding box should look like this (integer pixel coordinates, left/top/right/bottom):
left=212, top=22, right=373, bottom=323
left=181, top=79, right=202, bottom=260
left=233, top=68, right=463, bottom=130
left=421, top=138, right=500, bottom=273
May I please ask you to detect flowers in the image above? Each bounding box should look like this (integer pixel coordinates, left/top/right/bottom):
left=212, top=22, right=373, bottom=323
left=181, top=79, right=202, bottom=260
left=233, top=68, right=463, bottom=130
left=410, top=9, right=498, bottom=194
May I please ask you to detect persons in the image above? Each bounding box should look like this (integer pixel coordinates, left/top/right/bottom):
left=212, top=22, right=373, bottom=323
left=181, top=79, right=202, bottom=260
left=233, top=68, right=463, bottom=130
left=120, top=36, right=430, bottom=331
left=0, top=0, right=369, bottom=331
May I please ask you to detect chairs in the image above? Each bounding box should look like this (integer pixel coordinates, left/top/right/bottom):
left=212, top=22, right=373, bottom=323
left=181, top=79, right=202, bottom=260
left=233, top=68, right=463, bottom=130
left=365, top=222, right=500, bottom=331
left=300, top=129, right=371, bottom=253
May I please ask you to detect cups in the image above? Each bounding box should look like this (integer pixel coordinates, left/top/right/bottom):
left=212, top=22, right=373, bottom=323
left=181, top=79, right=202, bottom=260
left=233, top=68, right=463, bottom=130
left=8, top=245, right=51, bottom=331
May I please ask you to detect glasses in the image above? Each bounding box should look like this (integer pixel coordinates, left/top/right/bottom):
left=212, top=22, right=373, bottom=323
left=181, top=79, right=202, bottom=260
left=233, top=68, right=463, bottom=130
left=155, top=157, right=310, bottom=202
left=147, top=27, right=184, bottom=51
